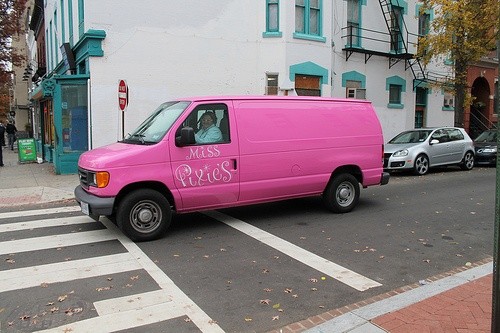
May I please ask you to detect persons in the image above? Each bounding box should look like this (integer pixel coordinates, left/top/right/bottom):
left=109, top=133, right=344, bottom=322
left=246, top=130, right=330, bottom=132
left=185, top=110, right=223, bottom=144
left=0, top=123, right=5, bottom=166
left=6, top=120, right=18, bottom=150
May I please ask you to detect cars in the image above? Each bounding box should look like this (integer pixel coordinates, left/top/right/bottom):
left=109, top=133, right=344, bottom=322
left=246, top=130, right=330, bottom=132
left=384, top=127, right=476, bottom=176
left=472, top=129, right=497, bottom=167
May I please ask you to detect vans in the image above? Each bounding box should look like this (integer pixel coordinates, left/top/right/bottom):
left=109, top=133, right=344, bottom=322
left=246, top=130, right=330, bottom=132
left=73, top=95, right=390, bottom=241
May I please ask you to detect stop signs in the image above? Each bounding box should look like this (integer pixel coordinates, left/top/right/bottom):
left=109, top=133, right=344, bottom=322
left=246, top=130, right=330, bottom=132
left=118, top=79, right=127, bottom=110
